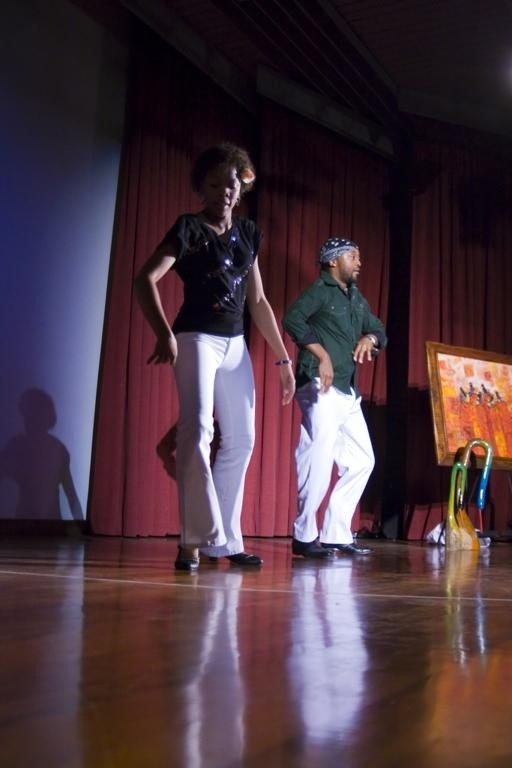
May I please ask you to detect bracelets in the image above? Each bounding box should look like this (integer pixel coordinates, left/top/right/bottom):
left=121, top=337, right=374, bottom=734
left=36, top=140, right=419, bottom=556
left=275, top=359, right=292, bottom=366
left=366, top=333, right=378, bottom=347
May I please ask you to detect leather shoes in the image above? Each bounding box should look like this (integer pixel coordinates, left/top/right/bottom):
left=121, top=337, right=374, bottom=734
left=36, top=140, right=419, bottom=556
left=292, top=538, right=336, bottom=559
left=174, top=543, right=201, bottom=572
left=320, top=541, right=376, bottom=555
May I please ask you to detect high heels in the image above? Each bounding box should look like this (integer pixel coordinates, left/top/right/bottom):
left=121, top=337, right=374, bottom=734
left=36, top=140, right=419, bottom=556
left=208, top=549, right=264, bottom=566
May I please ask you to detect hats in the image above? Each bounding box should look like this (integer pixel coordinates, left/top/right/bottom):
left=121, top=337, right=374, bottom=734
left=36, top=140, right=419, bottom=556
left=318, top=236, right=360, bottom=264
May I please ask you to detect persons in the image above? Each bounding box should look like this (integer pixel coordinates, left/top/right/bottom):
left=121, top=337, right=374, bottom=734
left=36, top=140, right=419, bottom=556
left=134, top=145, right=297, bottom=571
left=286, top=560, right=366, bottom=766
left=279, top=236, right=390, bottom=559
left=173, top=570, right=246, bottom=768
